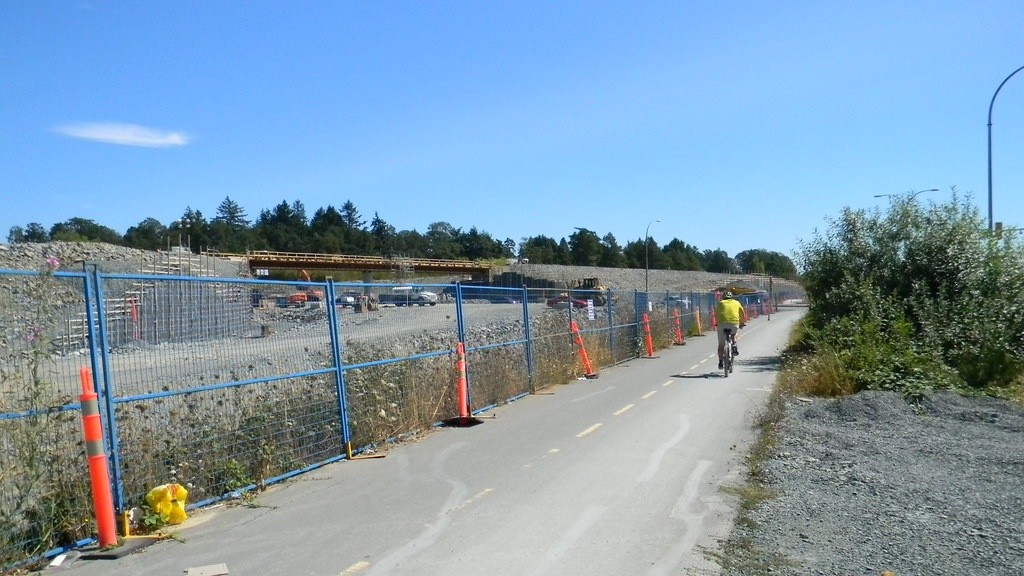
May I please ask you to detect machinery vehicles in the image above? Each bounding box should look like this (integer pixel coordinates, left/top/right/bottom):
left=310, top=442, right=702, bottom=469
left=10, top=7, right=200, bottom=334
left=563, top=278, right=618, bottom=308
left=275, top=292, right=308, bottom=308
left=299, top=269, right=324, bottom=301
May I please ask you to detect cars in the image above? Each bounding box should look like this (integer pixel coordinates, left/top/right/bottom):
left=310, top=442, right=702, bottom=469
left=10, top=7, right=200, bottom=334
left=490, top=297, right=517, bottom=304
left=737, top=291, right=770, bottom=306
left=656, top=296, right=691, bottom=309
left=335, top=292, right=361, bottom=308
left=546, top=295, right=587, bottom=310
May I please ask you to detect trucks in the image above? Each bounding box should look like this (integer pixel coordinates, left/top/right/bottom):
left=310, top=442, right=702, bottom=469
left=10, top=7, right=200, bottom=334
left=380, top=287, right=438, bottom=307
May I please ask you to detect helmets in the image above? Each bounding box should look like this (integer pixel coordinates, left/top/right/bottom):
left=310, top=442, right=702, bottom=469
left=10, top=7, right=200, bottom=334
left=722, top=292, right=732, bottom=298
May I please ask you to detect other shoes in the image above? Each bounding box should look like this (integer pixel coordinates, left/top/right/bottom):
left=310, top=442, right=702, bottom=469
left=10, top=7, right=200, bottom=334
left=732, top=346, right=739, bottom=356
left=719, top=360, right=723, bottom=368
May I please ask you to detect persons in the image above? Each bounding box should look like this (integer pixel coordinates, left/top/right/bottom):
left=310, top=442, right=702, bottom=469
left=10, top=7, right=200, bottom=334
left=714, top=291, right=745, bottom=367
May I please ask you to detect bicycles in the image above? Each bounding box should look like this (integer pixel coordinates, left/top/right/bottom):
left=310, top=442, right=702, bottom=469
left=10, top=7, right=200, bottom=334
left=715, top=323, right=747, bottom=377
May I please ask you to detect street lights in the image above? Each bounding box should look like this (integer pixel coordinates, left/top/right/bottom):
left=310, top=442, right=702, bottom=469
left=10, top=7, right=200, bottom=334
left=643, top=220, right=661, bottom=309
